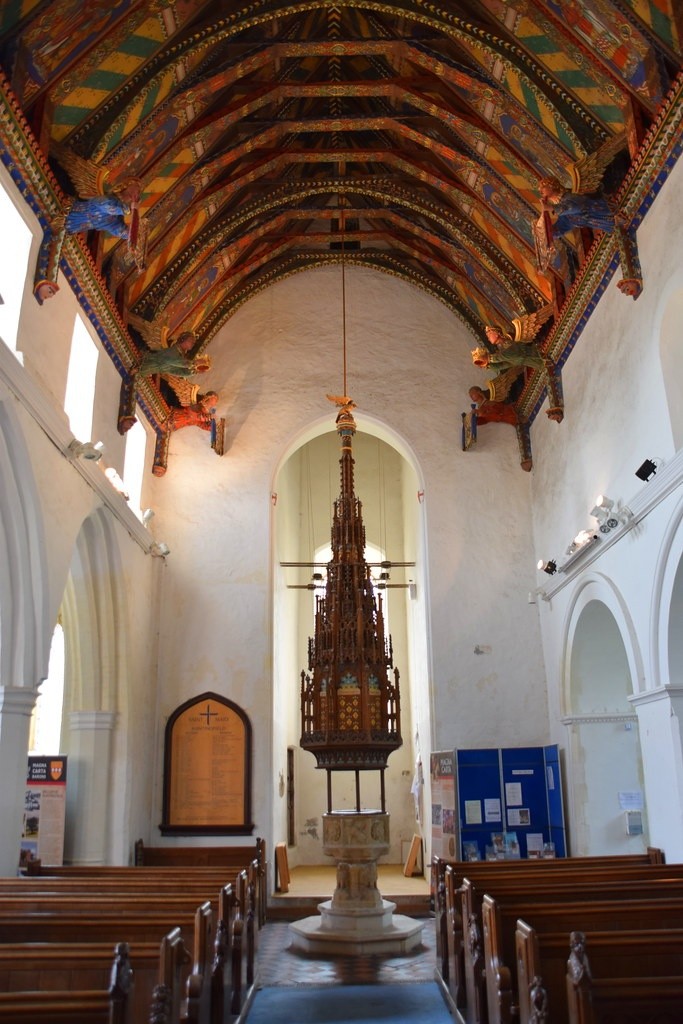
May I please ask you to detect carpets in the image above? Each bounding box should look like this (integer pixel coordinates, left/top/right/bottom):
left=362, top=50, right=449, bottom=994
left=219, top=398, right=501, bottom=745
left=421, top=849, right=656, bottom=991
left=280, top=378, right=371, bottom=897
left=245, top=982, right=455, bottom=1024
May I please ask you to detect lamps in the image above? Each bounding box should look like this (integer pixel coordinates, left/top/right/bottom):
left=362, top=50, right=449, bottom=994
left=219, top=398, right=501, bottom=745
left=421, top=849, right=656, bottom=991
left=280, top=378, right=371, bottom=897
left=159, top=542, right=170, bottom=556
left=70, top=439, right=103, bottom=462
left=635, top=459, right=657, bottom=482
left=301, top=194, right=403, bottom=770
left=143, top=509, right=155, bottom=524
left=566, top=495, right=619, bottom=554
left=537, top=560, right=557, bottom=575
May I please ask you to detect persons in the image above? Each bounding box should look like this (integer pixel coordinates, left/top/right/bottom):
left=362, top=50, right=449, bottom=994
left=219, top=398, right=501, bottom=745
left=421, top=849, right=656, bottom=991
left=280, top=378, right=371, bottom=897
left=486, top=326, right=544, bottom=371
left=138, top=331, right=205, bottom=377
left=468, top=386, right=517, bottom=426
left=169, top=391, right=219, bottom=431
left=65, top=174, right=143, bottom=239
left=538, top=176, right=615, bottom=236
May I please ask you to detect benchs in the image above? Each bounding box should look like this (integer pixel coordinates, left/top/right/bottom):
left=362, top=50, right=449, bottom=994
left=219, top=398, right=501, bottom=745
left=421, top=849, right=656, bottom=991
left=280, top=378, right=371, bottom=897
left=0, top=837, right=267, bottom=1024
left=432, top=847, right=683, bottom=1024
